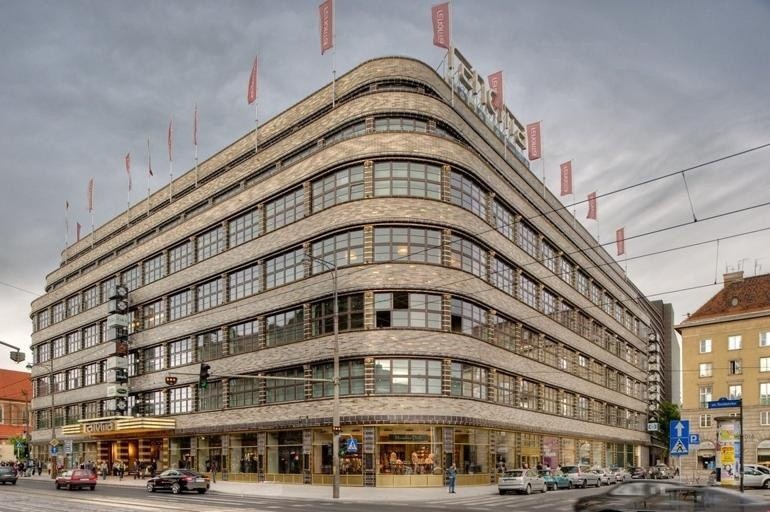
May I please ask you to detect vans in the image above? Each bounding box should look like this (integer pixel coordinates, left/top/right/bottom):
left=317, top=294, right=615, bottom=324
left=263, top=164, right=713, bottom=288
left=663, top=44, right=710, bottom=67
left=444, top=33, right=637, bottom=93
left=560, top=464, right=601, bottom=487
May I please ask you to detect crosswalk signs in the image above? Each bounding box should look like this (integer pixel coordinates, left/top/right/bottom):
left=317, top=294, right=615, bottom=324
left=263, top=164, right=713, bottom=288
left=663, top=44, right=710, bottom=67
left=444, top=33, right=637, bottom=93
left=346, top=439, right=359, bottom=453
left=671, top=437, right=689, bottom=456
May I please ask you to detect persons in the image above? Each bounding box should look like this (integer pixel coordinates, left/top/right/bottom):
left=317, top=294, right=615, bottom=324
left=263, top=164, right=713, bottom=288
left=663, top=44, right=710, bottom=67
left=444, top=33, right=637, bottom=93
left=208, top=460, right=219, bottom=484
left=545, top=463, right=551, bottom=476
left=555, top=463, right=562, bottom=476
left=535, top=461, right=543, bottom=477
left=13, top=457, right=158, bottom=481
left=447, top=461, right=459, bottom=494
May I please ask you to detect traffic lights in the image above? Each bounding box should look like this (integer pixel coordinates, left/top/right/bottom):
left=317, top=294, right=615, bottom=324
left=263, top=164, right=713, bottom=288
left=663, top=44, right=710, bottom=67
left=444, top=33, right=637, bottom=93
left=200, top=363, right=210, bottom=387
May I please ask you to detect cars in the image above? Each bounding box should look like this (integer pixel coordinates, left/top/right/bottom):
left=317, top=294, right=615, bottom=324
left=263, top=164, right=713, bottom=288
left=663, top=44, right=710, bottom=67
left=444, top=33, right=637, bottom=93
left=710, top=463, right=770, bottom=489
left=500, top=470, right=548, bottom=494
left=593, top=467, right=618, bottom=485
left=538, top=469, right=572, bottom=490
left=147, top=469, right=211, bottom=494
left=610, top=463, right=674, bottom=481
left=0, top=462, right=18, bottom=484
left=56, top=469, right=96, bottom=490
